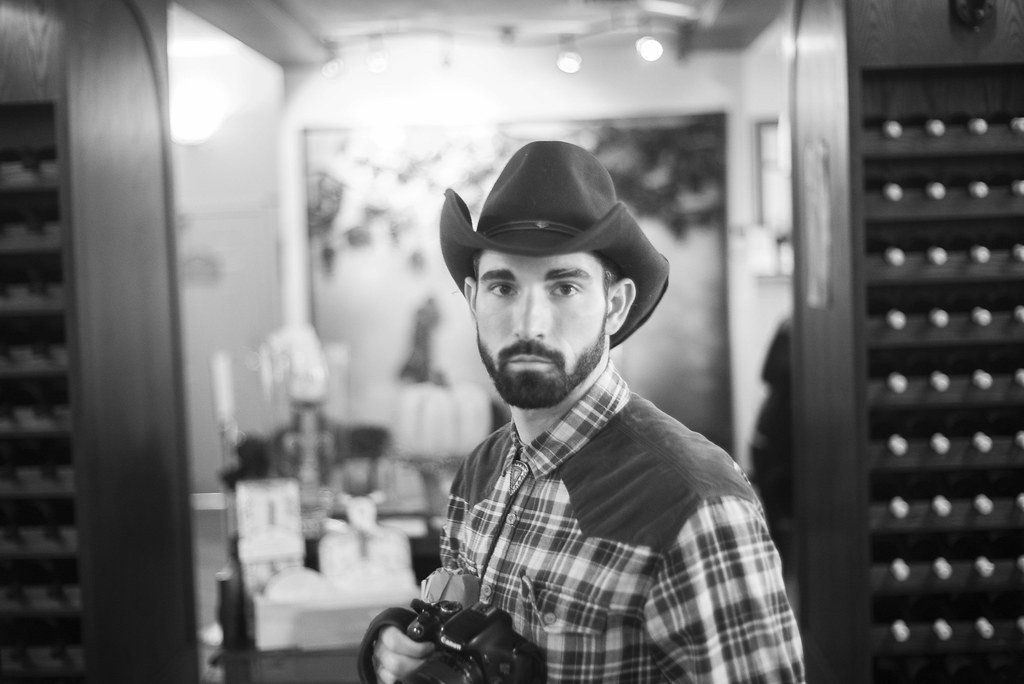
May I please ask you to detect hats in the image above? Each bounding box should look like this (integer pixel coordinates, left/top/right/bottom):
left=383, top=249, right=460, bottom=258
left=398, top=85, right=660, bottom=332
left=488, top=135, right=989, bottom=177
left=439, top=142, right=669, bottom=352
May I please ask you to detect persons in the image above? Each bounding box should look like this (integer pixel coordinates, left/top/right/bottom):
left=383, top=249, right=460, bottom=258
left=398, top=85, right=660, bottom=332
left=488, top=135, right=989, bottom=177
left=357, top=141, right=806, bottom=684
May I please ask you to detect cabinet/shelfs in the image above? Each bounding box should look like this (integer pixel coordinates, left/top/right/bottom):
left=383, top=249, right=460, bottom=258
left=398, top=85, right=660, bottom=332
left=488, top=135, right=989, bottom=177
left=793, top=0, right=1024, bottom=684
left=0, top=0, right=197, bottom=684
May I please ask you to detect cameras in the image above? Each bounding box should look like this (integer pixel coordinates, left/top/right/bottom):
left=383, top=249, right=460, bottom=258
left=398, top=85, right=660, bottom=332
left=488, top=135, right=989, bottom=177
left=390, top=600, right=546, bottom=684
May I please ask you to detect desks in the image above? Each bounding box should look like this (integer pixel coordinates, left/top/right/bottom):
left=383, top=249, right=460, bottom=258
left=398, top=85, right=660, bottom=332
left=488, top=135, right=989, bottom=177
left=324, top=459, right=446, bottom=538
